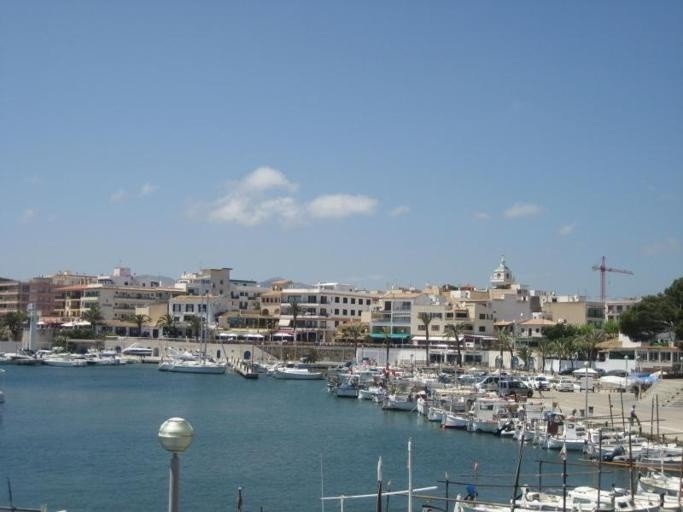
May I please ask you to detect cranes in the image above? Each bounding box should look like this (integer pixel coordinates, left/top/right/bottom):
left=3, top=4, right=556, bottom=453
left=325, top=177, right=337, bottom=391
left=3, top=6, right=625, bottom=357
left=590, top=255, right=633, bottom=302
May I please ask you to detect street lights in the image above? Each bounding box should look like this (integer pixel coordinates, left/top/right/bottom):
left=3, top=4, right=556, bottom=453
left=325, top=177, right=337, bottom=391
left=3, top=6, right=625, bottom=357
left=157, top=417, right=194, bottom=512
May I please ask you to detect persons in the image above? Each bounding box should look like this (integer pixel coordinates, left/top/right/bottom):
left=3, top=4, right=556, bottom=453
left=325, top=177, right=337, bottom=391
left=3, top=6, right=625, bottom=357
left=631, top=405, right=640, bottom=426
